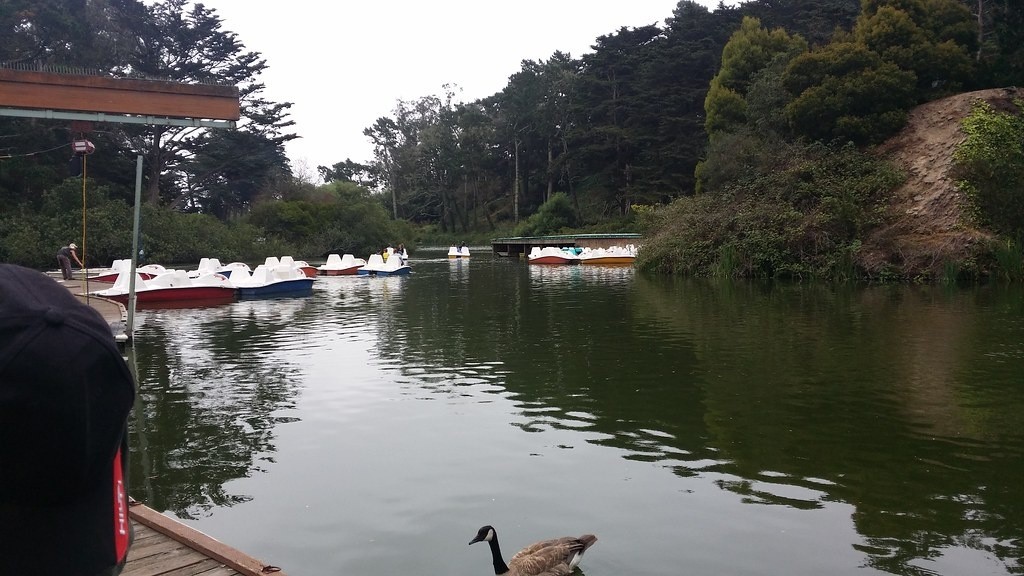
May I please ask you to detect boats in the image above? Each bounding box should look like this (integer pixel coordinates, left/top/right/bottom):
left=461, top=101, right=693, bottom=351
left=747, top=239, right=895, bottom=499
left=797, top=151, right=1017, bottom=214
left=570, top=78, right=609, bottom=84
left=227, top=263, right=318, bottom=297
left=448, top=246, right=471, bottom=260
left=315, top=254, right=367, bottom=276
left=357, top=253, right=412, bottom=276
left=89, top=253, right=319, bottom=283
left=528, top=246, right=581, bottom=264
left=80, top=270, right=238, bottom=302
left=382, top=248, right=409, bottom=260
left=581, top=245, right=636, bottom=264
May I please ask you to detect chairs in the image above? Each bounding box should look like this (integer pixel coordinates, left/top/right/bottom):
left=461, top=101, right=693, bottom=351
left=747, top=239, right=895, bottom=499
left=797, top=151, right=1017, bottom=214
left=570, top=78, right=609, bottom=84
left=448, top=246, right=470, bottom=255
left=528, top=244, right=639, bottom=257
left=100, top=248, right=408, bottom=289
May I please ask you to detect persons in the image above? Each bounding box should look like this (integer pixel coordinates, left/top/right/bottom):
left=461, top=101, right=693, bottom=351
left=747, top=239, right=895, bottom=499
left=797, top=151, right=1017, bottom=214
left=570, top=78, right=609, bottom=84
left=56, top=243, right=83, bottom=281
left=0, top=263, right=136, bottom=576
left=451, top=240, right=468, bottom=253
left=377, top=243, right=404, bottom=266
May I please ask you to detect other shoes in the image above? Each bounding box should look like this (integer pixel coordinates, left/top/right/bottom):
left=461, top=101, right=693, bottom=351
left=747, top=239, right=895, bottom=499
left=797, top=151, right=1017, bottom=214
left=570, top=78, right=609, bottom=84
left=67, top=278, right=75, bottom=280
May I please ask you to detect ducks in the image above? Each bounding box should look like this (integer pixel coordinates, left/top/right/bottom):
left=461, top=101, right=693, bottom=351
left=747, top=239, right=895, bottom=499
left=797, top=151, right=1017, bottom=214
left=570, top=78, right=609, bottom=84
left=468, top=524, right=598, bottom=576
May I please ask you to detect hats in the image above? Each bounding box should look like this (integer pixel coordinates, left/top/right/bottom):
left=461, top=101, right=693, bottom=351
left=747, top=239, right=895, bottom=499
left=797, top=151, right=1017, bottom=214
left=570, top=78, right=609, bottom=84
left=69, top=243, right=78, bottom=249
left=0, top=263, right=136, bottom=576
